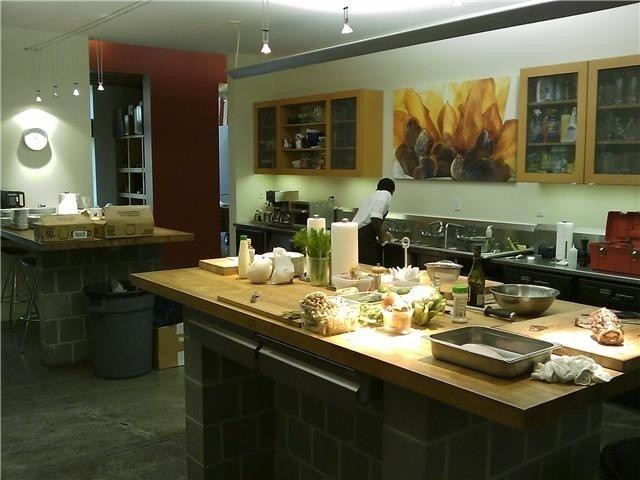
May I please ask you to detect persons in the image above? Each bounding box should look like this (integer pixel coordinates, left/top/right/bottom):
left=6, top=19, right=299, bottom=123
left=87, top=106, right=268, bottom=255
left=351, top=177, right=396, bottom=268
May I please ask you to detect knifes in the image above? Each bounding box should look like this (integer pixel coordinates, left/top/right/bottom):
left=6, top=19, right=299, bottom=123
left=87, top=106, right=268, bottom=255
left=250, top=290, right=260, bottom=303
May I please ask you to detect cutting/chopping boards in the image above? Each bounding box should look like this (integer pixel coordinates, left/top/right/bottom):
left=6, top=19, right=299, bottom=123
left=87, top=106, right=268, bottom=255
left=490, top=305, right=640, bottom=373
left=196, top=256, right=238, bottom=276
left=218, top=283, right=337, bottom=331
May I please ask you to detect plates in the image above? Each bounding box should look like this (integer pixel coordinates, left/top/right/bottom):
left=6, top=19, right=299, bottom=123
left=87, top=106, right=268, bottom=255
left=0, top=207, right=56, bottom=232
left=264, top=248, right=306, bottom=278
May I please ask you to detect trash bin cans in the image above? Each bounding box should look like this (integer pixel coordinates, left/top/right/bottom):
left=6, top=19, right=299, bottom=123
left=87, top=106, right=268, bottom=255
left=599, top=438, right=640, bottom=480
left=83, top=280, right=155, bottom=380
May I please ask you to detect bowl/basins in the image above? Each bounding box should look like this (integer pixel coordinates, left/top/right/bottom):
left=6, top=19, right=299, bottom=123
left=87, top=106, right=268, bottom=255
left=333, top=273, right=374, bottom=292
left=463, top=236, right=496, bottom=253
left=290, top=158, right=312, bottom=169
left=424, top=259, right=463, bottom=285
left=490, top=283, right=560, bottom=318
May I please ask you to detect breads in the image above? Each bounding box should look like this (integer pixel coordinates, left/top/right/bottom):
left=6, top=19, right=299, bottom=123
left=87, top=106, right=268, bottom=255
left=588, top=308, right=624, bottom=345
left=576, top=316, right=595, bottom=328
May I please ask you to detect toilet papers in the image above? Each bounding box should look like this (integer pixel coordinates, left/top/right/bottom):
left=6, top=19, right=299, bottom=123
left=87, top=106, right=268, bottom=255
left=555, top=219, right=575, bottom=261
left=305, top=214, right=326, bottom=263
left=329, top=218, right=359, bottom=286
left=55, top=192, right=78, bottom=215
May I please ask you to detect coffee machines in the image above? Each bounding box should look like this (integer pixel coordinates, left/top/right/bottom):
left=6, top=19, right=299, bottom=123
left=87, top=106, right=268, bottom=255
left=264, top=190, right=299, bottom=227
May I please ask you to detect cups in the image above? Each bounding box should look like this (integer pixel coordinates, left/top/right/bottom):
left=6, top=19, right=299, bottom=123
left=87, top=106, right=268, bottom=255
left=89, top=207, right=103, bottom=221
left=384, top=306, right=414, bottom=334
left=308, top=254, right=329, bottom=286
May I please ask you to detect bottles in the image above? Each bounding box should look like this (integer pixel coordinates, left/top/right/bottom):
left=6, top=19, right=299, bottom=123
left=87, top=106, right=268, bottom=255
left=238, top=235, right=249, bottom=278
left=247, top=238, right=255, bottom=265
left=468, top=243, right=486, bottom=309
left=450, top=284, right=470, bottom=324
left=568, top=243, right=578, bottom=268
left=254, top=209, right=264, bottom=223
left=546, top=107, right=560, bottom=142
left=486, top=225, right=494, bottom=238
left=565, top=107, right=577, bottom=143
left=429, top=271, right=441, bottom=294
left=579, top=237, right=590, bottom=268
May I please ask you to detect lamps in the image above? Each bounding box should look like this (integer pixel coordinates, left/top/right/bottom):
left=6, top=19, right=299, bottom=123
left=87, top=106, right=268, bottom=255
left=259, top=0, right=273, bottom=55
left=31, top=19, right=110, bottom=103
left=341, top=5, right=355, bottom=34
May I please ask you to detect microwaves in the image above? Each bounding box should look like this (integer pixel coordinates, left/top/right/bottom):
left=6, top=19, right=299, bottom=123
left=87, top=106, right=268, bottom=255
left=291, top=199, right=335, bottom=230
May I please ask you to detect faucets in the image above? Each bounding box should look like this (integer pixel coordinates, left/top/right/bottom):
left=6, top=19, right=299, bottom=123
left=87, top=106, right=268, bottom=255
left=444, top=222, right=466, bottom=230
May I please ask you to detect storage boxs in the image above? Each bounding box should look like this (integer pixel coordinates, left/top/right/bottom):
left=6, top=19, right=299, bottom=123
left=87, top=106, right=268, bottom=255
left=586, top=210, right=640, bottom=277
left=153, top=320, right=185, bottom=371
left=33, top=208, right=95, bottom=243
left=93, top=206, right=156, bottom=239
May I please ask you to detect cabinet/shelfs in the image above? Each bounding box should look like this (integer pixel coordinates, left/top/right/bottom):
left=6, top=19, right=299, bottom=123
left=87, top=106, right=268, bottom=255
left=114, top=134, right=147, bottom=204
left=516, top=53, right=639, bottom=186
left=252, top=87, right=383, bottom=179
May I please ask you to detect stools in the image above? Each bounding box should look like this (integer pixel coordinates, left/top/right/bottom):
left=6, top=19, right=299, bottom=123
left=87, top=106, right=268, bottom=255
left=0, top=246, right=41, bottom=333
left=18, top=256, right=40, bottom=351
